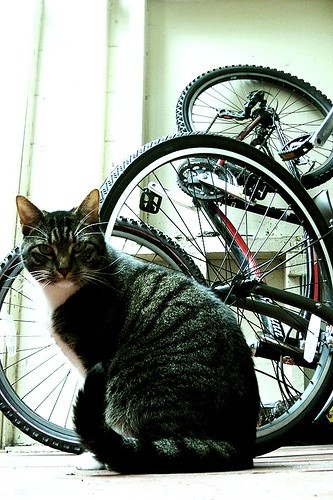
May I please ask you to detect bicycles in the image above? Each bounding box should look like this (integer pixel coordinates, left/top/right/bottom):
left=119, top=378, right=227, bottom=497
left=97, top=62, right=333, bottom=457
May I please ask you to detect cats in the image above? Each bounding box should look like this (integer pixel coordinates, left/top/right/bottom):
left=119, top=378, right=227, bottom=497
left=15, top=189, right=261, bottom=475
